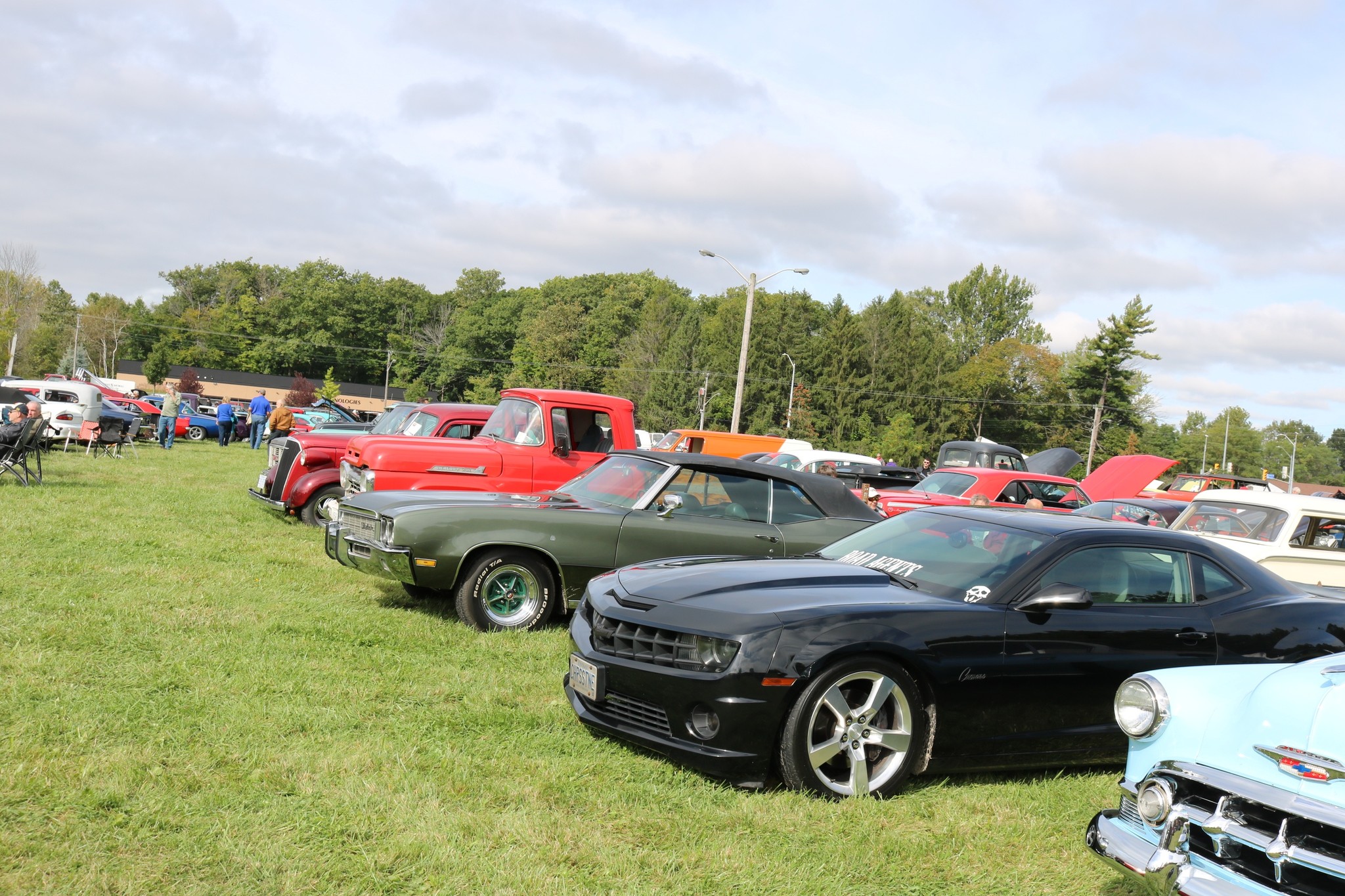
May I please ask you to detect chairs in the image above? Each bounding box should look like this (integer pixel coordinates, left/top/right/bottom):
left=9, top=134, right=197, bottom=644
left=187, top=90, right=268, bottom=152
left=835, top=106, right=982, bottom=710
left=673, top=493, right=703, bottom=515
left=710, top=502, right=749, bottom=520
left=1091, top=558, right=1142, bottom=603
left=576, top=424, right=604, bottom=452
left=0, top=411, right=63, bottom=488
left=594, top=429, right=613, bottom=453
left=64, top=415, right=143, bottom=459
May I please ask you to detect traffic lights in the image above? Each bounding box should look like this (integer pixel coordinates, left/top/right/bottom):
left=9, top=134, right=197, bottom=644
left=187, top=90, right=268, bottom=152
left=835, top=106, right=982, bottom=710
left=1214, top=464, right=1220, bottom=474
left=1227, top=463, right=1233, bottom=474
left=1262, top=470, right=1268, bottom=481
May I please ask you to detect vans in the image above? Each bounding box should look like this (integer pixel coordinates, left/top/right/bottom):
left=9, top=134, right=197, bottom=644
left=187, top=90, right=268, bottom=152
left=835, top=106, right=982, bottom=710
left=640, top=430, right=812, bottom=505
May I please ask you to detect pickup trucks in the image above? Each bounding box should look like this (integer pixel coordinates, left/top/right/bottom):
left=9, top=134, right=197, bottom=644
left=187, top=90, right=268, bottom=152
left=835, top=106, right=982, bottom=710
left=334, top=387, right=649, bottom=501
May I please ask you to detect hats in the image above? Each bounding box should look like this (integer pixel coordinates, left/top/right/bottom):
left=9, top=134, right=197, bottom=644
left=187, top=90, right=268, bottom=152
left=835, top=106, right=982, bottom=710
left=865, top=487, right=881, bottom=498
left=215, top=402, right=217, bottom=405
left=5, top=402, right=29, bottom=416
left=256, top=389, right=265, bottom=395
left=207, top=400, right=210, bottom=402
left=889, top=459, right=893, bottom=462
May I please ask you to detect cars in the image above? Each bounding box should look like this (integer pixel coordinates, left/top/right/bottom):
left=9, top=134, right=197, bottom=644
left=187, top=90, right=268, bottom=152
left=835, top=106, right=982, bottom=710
left=1085, top=652, right=1345, bottom=896
left=831, top=436, right=1345, bottom=591
left=246, top=402, right=878, bottom=531
left=564, top=507, right=1345, bottom=803
left=326, top=448, right=997, bottom=635
left=0, top=374, right=341, bottom=451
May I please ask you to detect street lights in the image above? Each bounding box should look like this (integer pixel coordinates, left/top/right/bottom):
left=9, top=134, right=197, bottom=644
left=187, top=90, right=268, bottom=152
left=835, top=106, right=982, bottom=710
left=1275, top=434, right=1296, bottom=494
left=782, top=353, right=795, bottom=429
left=1085, top=408, right=1112, bottom=477
left=1276, top=446, right=1292, bottom=493
left=699, top=249, right=809, bottom=434
left=702, top=393, right=720, bottom=431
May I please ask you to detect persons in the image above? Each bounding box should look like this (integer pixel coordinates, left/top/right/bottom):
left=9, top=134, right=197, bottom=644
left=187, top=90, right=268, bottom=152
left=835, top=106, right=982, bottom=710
left=158, top=383, right=182, bottom=449
left=982, top=498, right=1043, bottom=557
left=352, top=410, right=360, bottom=418
left=951, top=493, right=989, bottom=549
left=214, top=401, right=222, bottom=407
left=1292, top=487, right=1301, bottom=495
left=215, top=396, right=239, bottom=446
left=206, top=398, right=212, bottom=406
left=266, top=399, right=296, bottom=460
left=915, top=457, right=934, bottom=477
left=245, top=389, right=272, bottom=450
left=816, top=463, right=838, bottom=478
left=876, top=453, right=885, bottom=466
left=886, top=458, right=897, bottom=466
left=0, top=400, right=44, bottom=460
left=862, top=486, right=890, bottom=519
left=128, top=390, right=140, bottom=410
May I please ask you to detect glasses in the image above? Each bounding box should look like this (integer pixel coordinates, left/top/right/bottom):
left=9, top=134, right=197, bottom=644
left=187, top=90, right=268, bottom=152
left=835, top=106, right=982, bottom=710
left=924, top=462, right=928, bottom=463
left=868, top=497, right=879, bottom=502
left=8, top=409, right=18, bottom=413
left=877, top=456, right=880, bottom=457
